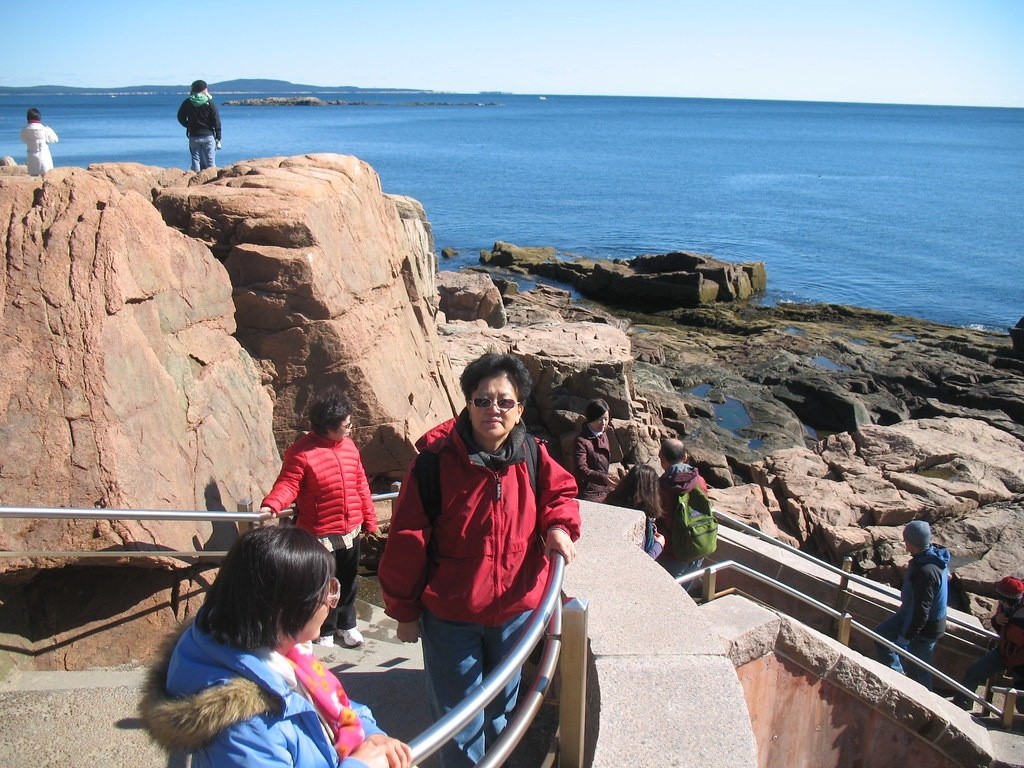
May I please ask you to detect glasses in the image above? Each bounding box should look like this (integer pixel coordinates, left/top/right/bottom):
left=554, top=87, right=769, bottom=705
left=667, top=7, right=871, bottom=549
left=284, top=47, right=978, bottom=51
left=339, top=421, right=352, bottom=429
left=326, top=576, right=341, bottom=609
left=469, top=398, right=521, bottom=408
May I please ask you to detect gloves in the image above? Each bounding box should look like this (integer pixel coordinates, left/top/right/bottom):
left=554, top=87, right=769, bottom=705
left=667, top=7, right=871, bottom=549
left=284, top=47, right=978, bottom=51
left=889, top=635, right=911, bottom=660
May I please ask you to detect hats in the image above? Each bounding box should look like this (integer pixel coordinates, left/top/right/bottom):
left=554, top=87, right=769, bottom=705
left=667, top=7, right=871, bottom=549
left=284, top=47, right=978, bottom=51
left=192, top=79, right=207, bottom=94
left=995, top=576, right=1024, bottom=600
left=903, top=520, right=930, bottom=551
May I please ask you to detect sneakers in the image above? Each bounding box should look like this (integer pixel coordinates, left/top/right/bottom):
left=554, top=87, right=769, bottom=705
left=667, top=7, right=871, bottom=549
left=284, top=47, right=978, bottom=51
left=335, top=627, right=364, bottom=648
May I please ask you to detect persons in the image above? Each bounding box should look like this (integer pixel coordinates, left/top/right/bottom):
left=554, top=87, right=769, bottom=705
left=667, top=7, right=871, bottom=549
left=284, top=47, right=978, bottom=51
left=601, top=465, right=666, bottom=561
left=954, top=577, right=1024, bottom=714
left=377, top=354, right=581, bottom=768
left=657, top=438, right=708, bottom=593
left=870, top=520, right=949, bottom=693
left=258, top=392, right=383, bottom=647
left=177, top=80, right=222, bottom=171
left=573, top=399, right=617, bottom=504
left=140, top=525, right=414, bottom=768
left=21, top=108, right=58, bottom=177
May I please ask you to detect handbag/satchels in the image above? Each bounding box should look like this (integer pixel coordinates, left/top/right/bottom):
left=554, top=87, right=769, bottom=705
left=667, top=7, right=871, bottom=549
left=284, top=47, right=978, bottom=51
left=359, top=536, right=388, bottom=572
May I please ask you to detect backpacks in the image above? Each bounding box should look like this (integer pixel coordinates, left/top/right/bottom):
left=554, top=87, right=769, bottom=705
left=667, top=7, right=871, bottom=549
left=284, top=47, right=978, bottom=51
left=670, top=482, right=718, bottom=562
